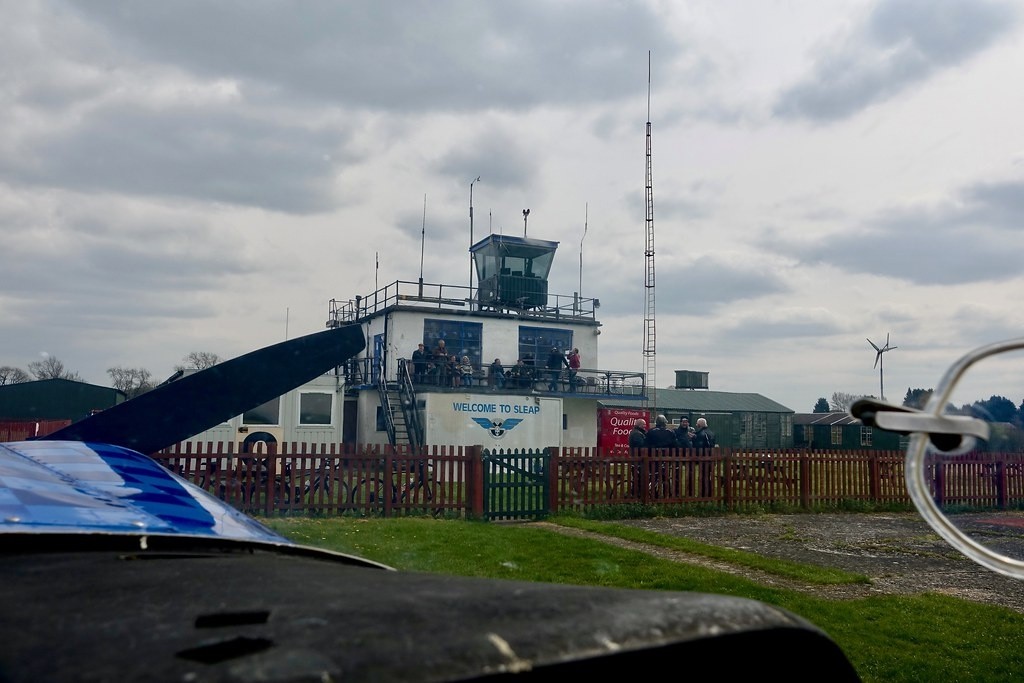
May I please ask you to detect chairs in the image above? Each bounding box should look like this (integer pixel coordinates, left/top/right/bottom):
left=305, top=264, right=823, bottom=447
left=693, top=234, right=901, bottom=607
left=574, top=375, right=603, bottom=392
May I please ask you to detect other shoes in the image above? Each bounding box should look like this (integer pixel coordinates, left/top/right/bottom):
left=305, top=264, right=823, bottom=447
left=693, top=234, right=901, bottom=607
left=554, top=386, right=557, bottom=391
left=548, top=385, right=552, bottom=391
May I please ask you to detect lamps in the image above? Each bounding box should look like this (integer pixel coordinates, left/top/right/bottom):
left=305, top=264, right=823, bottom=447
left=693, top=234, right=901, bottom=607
left=594, top=299, right=600, bottom=308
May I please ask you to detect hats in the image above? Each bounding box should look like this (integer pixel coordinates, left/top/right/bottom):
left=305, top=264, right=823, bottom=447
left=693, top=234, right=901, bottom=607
left=551, top=347, right=557, bottom=352
left=418, top=343, right=424, bottom=346
left=680, top=416, right=689, bottom=422
left=656, top=415, right=668, bottom=423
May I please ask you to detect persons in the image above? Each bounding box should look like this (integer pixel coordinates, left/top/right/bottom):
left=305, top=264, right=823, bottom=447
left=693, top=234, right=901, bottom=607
left=490, top=347, right=581, bottom=392
left=412, top=340, right=474, bottom=390
left=628, top=414, right=715, bottom=497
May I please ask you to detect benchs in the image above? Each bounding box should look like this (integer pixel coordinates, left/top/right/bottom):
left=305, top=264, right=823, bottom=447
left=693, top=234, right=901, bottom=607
left=715, top=476, right=800, bottom=502
left=407, top=373, right=571, bottom=390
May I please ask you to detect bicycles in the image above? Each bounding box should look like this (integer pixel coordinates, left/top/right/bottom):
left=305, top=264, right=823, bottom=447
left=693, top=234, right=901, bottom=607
left=352, top=453, right=445, bottom=518
left=249, top=457, right=350, bottom=517
left=167, top=462, right=246, bottom=511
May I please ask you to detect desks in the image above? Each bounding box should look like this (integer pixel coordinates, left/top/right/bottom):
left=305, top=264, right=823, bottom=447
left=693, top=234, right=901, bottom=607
left=729, top=464, right=790, bottom=476
left=602, top=376, right=625, bottom=394
